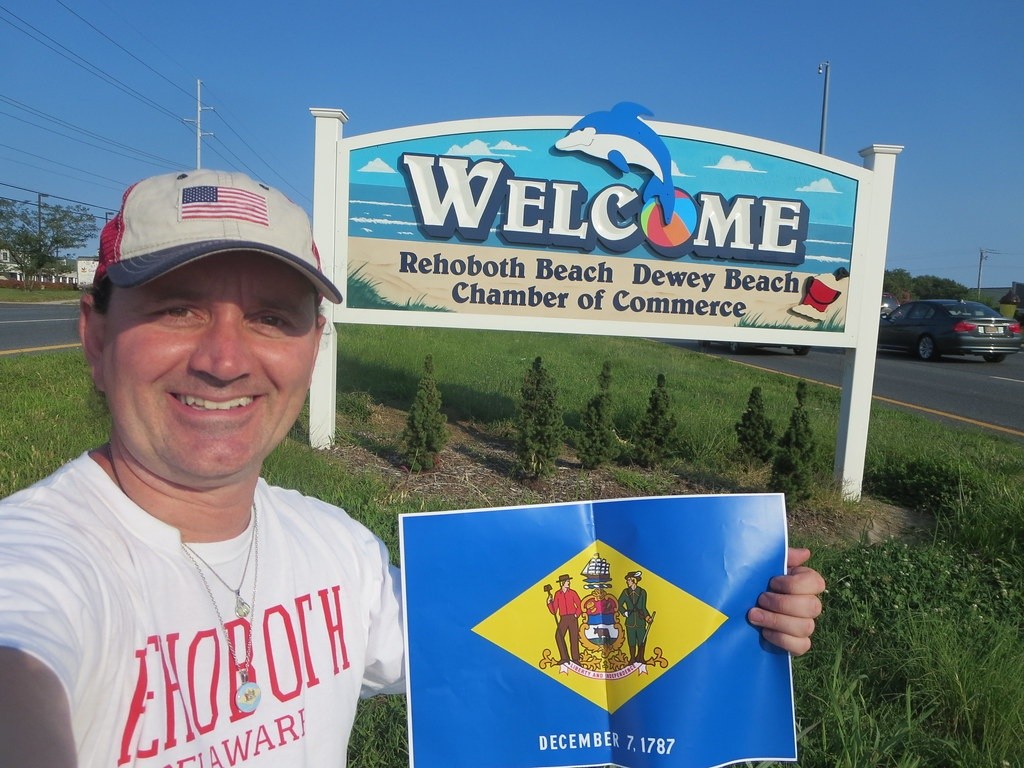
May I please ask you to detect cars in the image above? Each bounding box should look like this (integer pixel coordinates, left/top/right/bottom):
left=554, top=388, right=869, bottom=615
left=878, top=299, right=1024, bottom=363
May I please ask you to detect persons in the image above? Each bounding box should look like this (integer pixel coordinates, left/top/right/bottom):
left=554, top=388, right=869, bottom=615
left=0, top=169, right=825, bottom=768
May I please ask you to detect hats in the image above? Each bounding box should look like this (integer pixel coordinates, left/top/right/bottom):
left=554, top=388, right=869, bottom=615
left=95, top=169, right=343, bottom=304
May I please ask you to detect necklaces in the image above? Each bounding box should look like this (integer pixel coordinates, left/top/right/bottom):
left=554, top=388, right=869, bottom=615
left=107, top=444, right=261, bottom=712
